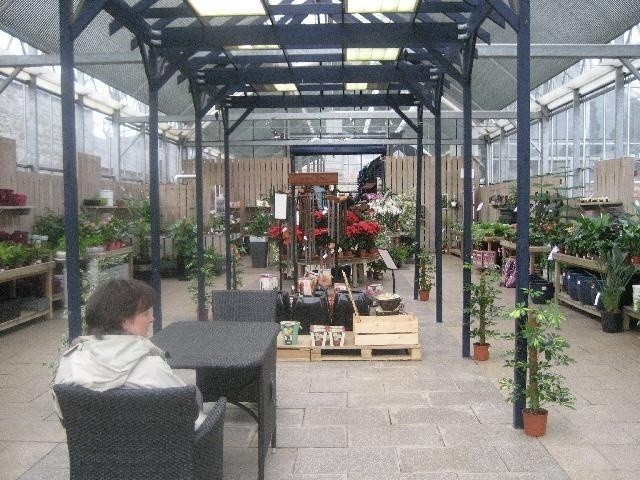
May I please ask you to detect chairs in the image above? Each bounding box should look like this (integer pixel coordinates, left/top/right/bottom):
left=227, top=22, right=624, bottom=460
left=53, top=381, right=226, bottom=480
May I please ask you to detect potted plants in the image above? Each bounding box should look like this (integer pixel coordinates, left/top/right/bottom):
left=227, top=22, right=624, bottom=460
left=462, top=258, right=514, bottom=363
left=1, top=181, right=228, bottom=316
left=410, top=241, right=443, bottom=304
left=497, top=282, right=579, bottom=437
left=582, top=245, right=637, bottom=333
left=464, top=182, right=640, bottom=307
left=249, top=188, right=415, bottom=281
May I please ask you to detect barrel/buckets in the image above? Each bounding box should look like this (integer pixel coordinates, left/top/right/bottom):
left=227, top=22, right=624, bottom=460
left=632, top=285, right=640, bottom=310
left=530, top=280, right=553, bottom=303
left=99, top=190, right=113, bottom=206
left=562, top=269, right=607, bottom=311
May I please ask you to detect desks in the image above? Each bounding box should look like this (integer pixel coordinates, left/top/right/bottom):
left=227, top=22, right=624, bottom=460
left=143, top=320, right=278, bottom=480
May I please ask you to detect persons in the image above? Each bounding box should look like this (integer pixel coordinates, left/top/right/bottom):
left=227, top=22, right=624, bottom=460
left=49, top=276, right=209, bottom=434
